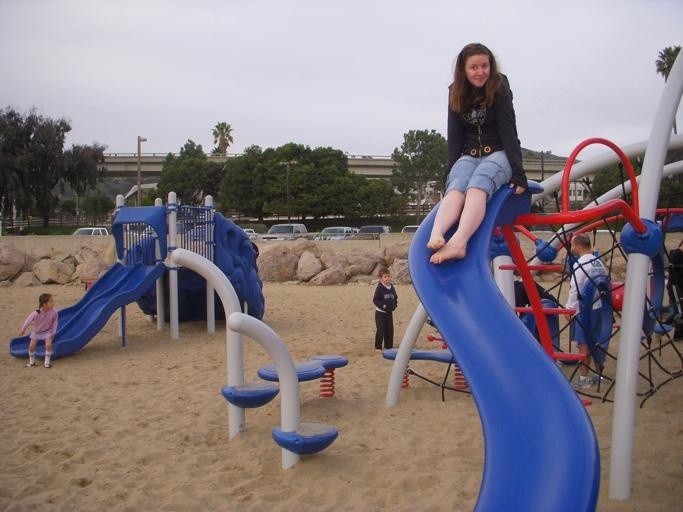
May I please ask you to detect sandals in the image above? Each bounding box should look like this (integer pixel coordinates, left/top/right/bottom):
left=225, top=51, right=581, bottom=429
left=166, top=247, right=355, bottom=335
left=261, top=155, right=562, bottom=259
left=25, top=362, right=37, bottom=368
left=44, top=363, right=51, bottom=369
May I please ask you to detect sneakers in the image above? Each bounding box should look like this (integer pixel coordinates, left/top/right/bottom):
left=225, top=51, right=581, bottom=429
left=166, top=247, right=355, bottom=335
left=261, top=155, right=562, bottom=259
left=590, top=372, right=608, bottom=385
left=571, top=377, right=592, bottom=389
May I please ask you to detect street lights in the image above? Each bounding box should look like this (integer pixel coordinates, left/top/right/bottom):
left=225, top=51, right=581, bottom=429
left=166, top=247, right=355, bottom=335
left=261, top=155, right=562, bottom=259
left=541, top=146, right=554, bottom=216
left=136, top=135, right=147, bottom=207
left=277, top=160, right=297, bottom=223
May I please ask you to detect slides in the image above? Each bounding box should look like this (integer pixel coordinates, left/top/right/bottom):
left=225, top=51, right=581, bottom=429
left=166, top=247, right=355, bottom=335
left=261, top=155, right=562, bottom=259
left=407, top=178, right=600, bottom=510
left=10, top=262, right=164, bottom=359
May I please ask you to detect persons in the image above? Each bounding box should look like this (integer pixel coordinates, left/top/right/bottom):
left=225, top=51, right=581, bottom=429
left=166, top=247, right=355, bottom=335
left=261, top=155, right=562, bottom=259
left=668, top=240, right=683, bottom=341
left=18, top=293, right=59, bottom=368
left=563, top=233, right=612, bottom=389
left=513, top=280, right=560, bottom=352
left=427, top=43, right=530, bottom=265
left=372, top=268, right=398, bottom=353
